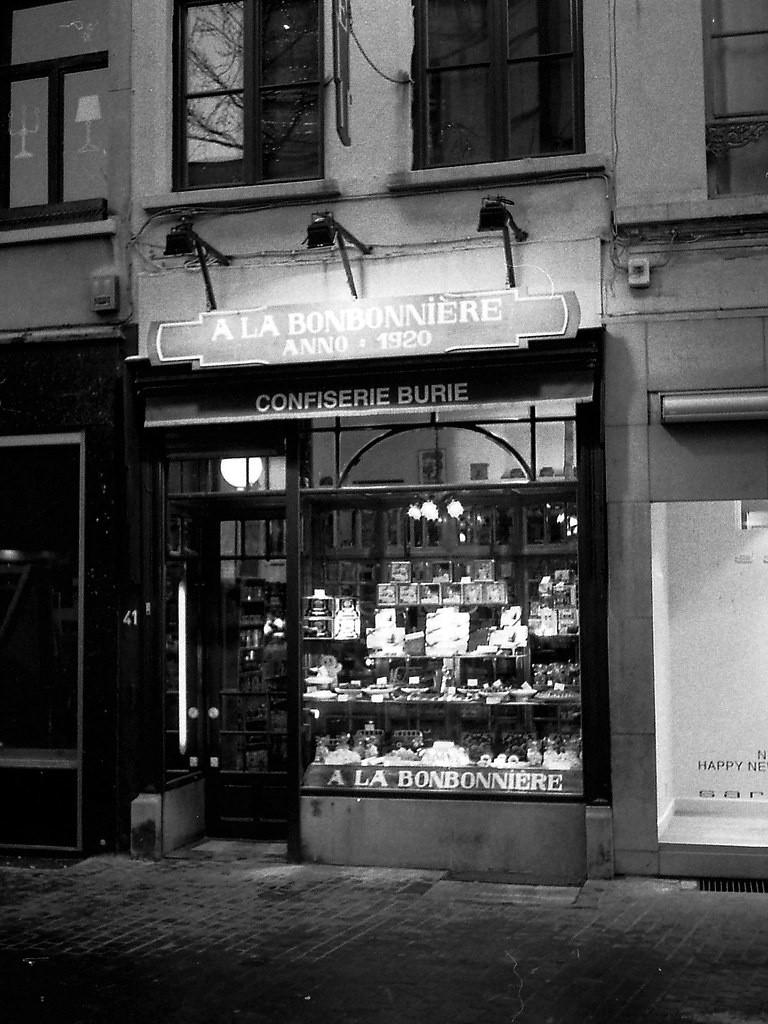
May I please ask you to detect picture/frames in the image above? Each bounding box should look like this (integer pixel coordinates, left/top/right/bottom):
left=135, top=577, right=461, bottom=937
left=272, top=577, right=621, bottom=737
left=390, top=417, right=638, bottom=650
left=418, top=447, right=446, bottom=486
left=470, top=463, right=489, bottom=482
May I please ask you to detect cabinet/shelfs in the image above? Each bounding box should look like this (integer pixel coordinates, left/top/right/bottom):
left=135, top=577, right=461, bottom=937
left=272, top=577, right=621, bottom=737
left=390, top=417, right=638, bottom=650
left=302, top=636, right=584, bottom=797
left=222, top=574, right=268, bottom=773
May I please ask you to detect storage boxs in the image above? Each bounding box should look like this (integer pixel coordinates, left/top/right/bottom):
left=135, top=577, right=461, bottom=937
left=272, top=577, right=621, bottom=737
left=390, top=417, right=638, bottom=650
left=301, top=559, right=581, bottom=636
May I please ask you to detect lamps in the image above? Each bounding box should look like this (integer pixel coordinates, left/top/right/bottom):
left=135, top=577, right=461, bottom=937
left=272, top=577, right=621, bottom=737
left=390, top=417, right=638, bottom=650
left=477, top=195, right=516, bottom=232
left=406, top=411, right=467, bottom=522
left=163, top=221, right=195, bottom=256
left=219, top=457, right=264, bottom=491
left=300, top=210, right=339, bottom=251
